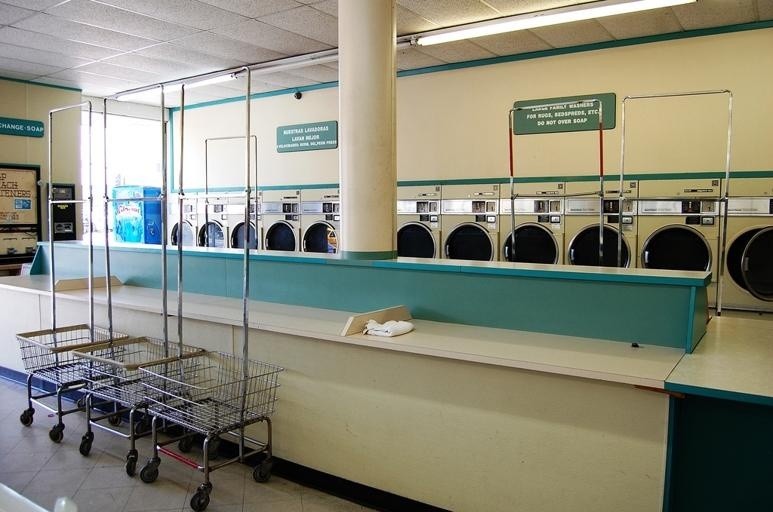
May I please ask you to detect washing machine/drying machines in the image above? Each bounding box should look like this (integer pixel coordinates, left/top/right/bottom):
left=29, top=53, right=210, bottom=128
left=160, top=193, right=773, bottom=316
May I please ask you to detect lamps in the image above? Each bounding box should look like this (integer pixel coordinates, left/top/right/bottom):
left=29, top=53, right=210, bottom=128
left=236, top=36, right=416, bottom=79
left=116, top=70, right=235, bottom=99
left=417, top=0, right=695, bottom=47
